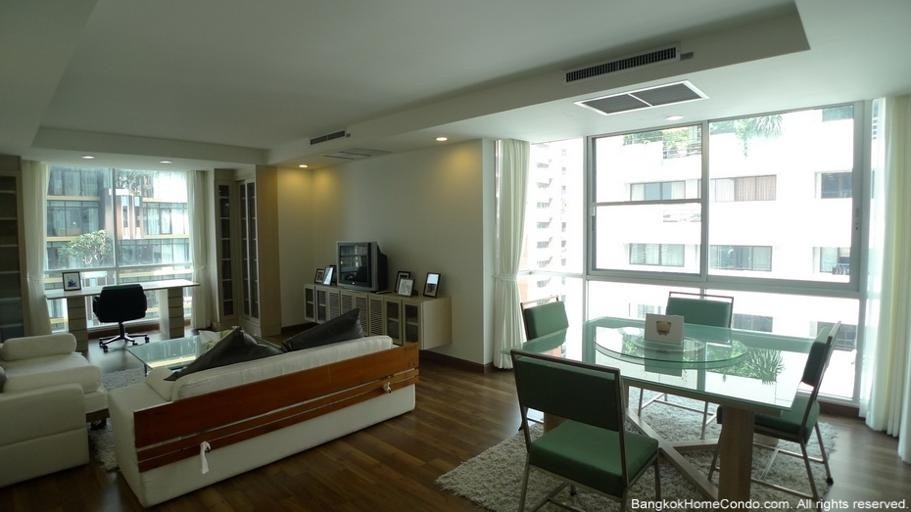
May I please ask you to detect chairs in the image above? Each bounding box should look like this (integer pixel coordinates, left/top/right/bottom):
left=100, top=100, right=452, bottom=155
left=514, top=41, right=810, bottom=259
left=93, top=284, right=149, bottom=351
left=510, top=291, right=841, bottom=512
left=0, top=333, right=109, bottom=488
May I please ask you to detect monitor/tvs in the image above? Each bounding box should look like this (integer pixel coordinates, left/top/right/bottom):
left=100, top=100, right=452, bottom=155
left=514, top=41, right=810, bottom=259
left=336, top=240, right=387, bottom=292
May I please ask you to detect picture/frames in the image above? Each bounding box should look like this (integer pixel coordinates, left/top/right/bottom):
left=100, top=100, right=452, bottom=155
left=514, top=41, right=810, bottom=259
left=314, top=265, right=336, bottom=286
left=395, top=271, right=441, bottom=298
left=63, top=271, right=81, bottom=291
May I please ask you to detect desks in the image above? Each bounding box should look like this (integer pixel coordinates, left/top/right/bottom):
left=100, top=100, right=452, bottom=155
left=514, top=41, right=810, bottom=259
left=45, top=279, right=200, bottom=352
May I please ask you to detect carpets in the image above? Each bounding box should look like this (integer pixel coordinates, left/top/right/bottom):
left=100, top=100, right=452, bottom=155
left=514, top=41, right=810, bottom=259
left=435, top=386, right=839, bottom=512
left=87, top=366, right=152, bottom=470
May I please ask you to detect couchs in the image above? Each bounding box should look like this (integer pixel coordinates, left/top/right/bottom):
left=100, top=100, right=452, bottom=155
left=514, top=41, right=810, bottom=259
left=107, top=335, right=419, bottom=507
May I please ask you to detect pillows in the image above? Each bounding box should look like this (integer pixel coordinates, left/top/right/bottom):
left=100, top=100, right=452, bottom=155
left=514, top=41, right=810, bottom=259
left=2, top=365, right=101, bottom=393
left=281, top=307, right=365, bottom=353
left=1, top=333, right=77, bottom=361
left=164, top=327, right=284, bottom=380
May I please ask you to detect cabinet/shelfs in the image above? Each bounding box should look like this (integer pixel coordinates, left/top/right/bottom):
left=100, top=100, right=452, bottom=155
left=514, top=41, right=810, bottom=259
left=304, top=283, right=451, bottom=350
left=204, top=177, right=281, bottom=337
left=0, top=170, right=31, bottom=343
